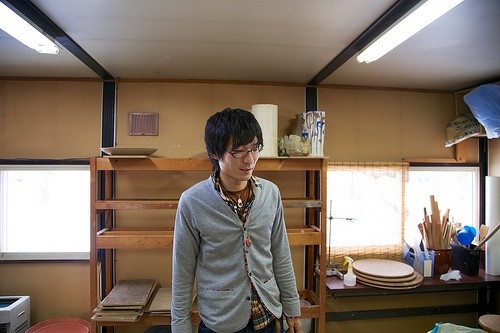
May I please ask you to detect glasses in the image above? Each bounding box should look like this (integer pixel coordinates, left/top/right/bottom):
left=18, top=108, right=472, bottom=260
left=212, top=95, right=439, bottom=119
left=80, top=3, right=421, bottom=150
left=224, top=145, right=264, bottom=159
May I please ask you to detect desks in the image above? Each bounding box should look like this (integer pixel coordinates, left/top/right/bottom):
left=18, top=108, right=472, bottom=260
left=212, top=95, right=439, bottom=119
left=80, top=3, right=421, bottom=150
left=326, top=271, right=500, bottom=330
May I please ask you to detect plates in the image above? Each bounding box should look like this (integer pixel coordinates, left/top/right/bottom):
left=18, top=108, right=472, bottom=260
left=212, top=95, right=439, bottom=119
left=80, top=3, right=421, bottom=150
left=99, top=147, right=157, bottom=155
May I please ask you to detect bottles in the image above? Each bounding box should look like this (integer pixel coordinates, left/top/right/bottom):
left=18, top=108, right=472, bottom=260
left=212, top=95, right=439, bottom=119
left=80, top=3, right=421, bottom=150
left=426, top=246, right=453, bottom=274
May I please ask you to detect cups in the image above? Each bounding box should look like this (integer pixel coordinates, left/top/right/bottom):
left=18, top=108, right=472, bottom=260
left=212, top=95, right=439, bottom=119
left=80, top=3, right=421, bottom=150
left=250, top=103, right=278, bottom=159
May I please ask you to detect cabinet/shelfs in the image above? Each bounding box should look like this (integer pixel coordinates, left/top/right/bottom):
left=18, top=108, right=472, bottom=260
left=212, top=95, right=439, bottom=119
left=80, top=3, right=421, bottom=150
left=453, top=82, right=500, bottom=137
left=89, top=156, right=330, bottom=333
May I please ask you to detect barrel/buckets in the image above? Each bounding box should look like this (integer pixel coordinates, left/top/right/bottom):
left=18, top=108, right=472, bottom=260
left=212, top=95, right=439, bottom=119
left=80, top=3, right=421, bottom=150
left=283, top=299, right=311, bottom=333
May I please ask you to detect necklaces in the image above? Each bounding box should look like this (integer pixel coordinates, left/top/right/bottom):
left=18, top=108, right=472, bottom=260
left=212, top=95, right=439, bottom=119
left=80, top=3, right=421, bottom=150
left=227, top=186, right=248, bottom=207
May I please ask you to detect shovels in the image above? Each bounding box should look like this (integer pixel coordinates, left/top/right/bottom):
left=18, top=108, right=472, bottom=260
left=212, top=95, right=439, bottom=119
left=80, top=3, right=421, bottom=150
left=480, top=223, right=489, bottom=241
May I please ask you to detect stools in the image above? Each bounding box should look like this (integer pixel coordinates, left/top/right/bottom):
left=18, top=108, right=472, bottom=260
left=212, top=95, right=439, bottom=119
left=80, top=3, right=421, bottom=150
left=478, top=314, right=500, bottom=333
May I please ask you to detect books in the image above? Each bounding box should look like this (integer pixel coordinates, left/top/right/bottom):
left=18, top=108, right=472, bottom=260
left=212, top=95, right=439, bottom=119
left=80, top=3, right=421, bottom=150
left=91, top=279, right=197, bottom=322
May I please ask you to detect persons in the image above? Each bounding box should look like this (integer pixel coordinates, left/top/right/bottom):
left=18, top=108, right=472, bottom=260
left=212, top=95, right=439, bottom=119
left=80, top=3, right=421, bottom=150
left=172, top=108, right=303, bottom=333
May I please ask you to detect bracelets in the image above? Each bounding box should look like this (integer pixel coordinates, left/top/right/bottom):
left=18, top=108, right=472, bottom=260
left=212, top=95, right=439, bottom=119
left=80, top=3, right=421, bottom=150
left=288, top=316, right=299, bottom=319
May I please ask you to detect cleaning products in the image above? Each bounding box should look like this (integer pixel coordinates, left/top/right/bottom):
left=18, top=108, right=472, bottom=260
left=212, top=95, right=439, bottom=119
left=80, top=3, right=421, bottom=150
left=342, top=255, right=357, bottom=287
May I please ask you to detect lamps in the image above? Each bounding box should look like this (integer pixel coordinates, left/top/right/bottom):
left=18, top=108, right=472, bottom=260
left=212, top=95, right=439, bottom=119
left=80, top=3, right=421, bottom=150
left=0, top=0, right=60, bottom=55
left=354, top=0, right=464, bottom=64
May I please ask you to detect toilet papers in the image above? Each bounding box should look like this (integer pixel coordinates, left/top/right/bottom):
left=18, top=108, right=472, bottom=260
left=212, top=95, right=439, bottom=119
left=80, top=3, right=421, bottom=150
left=250, top=103, right=278, bottom=159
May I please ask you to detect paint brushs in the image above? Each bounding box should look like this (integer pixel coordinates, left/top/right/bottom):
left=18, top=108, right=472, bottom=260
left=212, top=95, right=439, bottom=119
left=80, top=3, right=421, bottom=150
left=419, top=239, right=424, bottom=252
left=409, top=248, right=415, bottom=253
left=417, top=195, right=451, bottom=248
left=409, top=255, right=415, bottom=258
